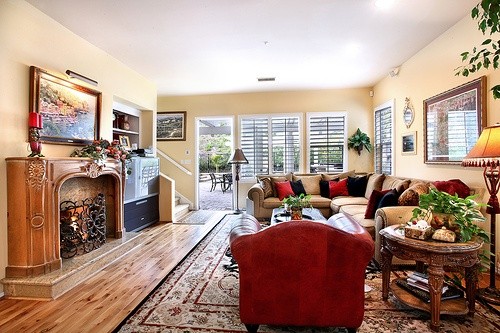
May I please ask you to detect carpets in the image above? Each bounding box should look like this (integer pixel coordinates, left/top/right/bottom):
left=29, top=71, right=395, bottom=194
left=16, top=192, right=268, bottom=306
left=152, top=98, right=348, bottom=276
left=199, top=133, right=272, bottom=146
left=174, top=211, right=215, bottom=224
left=111, top=214, right=500, bottom=333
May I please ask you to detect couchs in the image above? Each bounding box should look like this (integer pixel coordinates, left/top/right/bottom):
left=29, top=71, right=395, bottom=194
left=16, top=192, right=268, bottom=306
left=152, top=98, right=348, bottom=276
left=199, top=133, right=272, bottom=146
left=247, top=173, right=484, bottom=268
left=229, top=212, right=376, bottom=333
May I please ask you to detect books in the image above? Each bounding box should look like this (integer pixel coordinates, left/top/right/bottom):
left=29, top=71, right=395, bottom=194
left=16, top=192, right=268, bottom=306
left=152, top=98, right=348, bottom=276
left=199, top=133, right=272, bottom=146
left=395, top=274, right=461, bottom=303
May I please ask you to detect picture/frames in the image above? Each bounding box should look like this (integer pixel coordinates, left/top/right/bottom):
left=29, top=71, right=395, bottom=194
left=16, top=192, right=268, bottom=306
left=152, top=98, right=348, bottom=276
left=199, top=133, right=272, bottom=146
left=156, top=111, right=187, bottom=142
left=29, top=66, right=102, bottom=146
left=402, top=75, right=488, bottom=164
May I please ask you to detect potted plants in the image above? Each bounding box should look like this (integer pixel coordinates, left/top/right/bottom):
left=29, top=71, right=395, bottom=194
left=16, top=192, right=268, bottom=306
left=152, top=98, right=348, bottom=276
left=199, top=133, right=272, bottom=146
left=277, top=193, right=314, bottom=220
left=347, top=128, right=373, bottom=155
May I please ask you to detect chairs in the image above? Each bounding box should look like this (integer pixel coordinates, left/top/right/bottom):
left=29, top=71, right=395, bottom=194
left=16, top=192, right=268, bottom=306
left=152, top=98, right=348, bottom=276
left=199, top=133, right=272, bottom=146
left=209, top=173, right=231, bottom=194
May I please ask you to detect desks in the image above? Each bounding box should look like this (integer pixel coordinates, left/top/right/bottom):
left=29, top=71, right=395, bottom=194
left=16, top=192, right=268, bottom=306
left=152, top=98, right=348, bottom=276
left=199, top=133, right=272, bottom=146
left=379, top=224, right=484, bottom=329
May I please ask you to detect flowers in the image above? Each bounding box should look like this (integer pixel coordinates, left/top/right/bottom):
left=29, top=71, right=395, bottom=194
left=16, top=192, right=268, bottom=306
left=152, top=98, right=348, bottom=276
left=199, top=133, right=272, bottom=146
left=119, top=135, right=130, bottom=148
left=69, top=138, right=134, bottom=175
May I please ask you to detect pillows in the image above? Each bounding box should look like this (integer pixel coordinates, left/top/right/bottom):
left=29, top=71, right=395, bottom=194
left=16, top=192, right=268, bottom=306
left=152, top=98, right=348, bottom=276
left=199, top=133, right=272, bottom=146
left=256, top=174, right=470, bottom=219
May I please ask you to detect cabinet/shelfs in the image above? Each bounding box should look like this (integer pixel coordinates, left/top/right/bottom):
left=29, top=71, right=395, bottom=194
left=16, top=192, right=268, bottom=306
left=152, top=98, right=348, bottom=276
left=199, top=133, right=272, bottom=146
left=112, top=109, right=160, bottom=233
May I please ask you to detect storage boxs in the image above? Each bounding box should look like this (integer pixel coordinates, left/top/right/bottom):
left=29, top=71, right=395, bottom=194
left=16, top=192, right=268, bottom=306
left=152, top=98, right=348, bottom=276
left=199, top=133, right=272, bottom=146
left=405, top=225, right=431, bottom=240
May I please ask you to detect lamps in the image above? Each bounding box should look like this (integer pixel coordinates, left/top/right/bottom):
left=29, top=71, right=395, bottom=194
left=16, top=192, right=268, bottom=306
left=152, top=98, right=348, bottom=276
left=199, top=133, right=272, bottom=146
left=227, top=148, right=249, bottom=215
left=462, top=124, right=500, bottom=304
left=66, top=70, right=98, bottom=86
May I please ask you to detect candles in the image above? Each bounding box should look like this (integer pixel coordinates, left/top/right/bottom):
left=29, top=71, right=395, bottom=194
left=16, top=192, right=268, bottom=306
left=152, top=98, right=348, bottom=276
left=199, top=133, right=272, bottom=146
left=28, top=112, right=43, bottom=129
